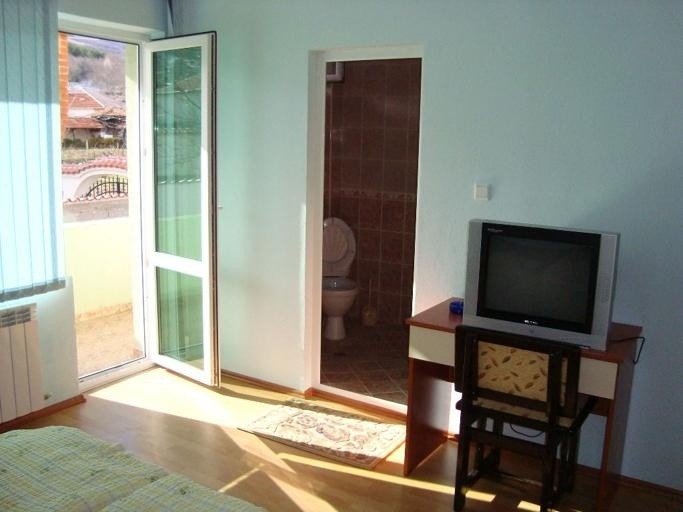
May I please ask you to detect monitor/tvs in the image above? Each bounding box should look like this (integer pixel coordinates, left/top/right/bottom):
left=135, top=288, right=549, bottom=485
left=463, top=218, right=621, bottom=353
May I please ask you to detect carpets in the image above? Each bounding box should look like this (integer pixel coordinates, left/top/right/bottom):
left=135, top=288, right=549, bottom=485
left=237, top=397, right=406, bottom=470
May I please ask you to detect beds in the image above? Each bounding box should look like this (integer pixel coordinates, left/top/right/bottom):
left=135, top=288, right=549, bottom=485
left=0, top=425, right=271, bottom=512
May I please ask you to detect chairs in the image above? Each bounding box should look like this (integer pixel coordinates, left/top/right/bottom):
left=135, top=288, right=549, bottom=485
left=454, top=324, right=590, bottom=512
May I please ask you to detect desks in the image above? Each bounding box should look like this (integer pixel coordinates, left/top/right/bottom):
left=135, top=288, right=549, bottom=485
left=403, top=297, right=643, bottom=512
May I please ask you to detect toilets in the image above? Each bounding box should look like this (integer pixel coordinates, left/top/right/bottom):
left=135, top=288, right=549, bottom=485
left=322, top=215, right=360, bottom=340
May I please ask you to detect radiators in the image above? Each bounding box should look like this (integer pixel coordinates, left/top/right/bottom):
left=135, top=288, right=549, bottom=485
left=0, top=303, right=43, bottom=425
